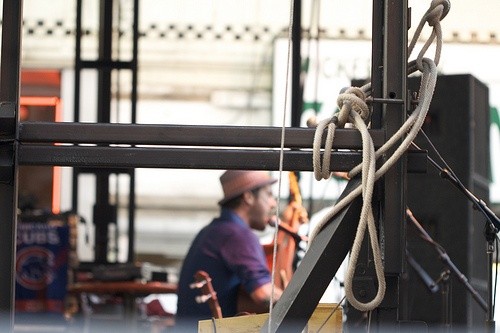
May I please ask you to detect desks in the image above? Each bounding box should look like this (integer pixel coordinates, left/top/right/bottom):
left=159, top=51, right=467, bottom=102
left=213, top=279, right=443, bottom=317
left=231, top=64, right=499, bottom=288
left=68, top=281, right=179, bottom=319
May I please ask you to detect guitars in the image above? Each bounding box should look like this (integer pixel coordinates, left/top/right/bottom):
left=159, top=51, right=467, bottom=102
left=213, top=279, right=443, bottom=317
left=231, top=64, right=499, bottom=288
left=190, top=270, right=223, bottom=319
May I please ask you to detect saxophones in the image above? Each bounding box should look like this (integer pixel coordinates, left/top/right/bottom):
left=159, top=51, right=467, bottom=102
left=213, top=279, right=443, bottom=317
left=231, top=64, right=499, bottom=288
left=269, top=221, right=311, bottom=273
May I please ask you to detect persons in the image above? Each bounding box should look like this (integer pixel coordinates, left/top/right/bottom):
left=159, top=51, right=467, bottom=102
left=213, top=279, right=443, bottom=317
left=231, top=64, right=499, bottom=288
left=176, top=170, right=288, bottom=333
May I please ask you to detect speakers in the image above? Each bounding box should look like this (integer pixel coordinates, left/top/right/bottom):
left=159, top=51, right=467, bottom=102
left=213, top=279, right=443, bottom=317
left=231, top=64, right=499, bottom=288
left=342, top=73, right=493, bottom=333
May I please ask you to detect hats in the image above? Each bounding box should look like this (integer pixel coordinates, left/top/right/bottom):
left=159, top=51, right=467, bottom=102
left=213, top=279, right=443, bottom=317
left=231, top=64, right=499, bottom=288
left=212, top=170, right=280, bottom=206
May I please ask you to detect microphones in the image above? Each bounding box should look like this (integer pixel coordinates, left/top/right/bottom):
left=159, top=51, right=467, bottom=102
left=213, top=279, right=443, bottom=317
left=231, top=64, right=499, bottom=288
left=268, top=215, right=308, bottom=243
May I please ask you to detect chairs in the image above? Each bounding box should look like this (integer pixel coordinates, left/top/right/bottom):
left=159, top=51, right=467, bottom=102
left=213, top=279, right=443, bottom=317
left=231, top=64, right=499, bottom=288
left=73, top=261, right=144, bottom=320
